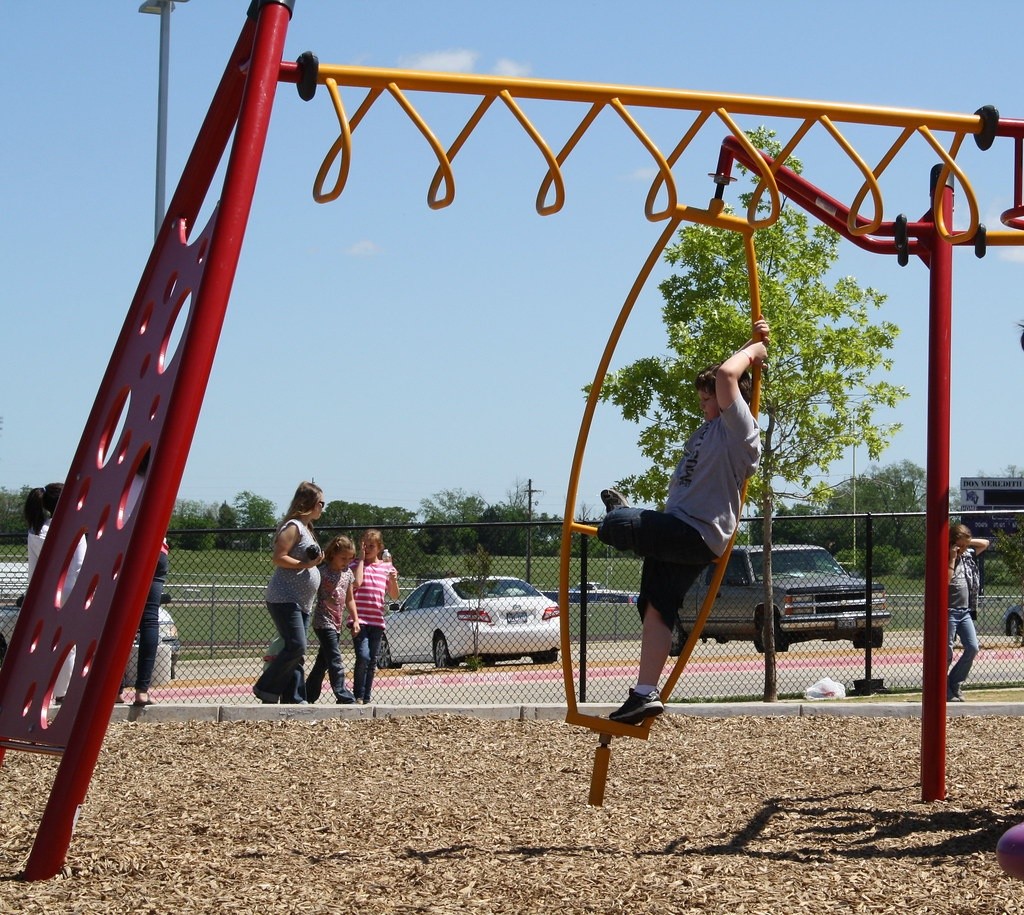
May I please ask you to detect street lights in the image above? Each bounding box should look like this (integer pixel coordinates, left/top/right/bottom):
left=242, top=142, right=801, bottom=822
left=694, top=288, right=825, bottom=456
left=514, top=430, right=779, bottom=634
left=140, top=0, right=175, bottom=242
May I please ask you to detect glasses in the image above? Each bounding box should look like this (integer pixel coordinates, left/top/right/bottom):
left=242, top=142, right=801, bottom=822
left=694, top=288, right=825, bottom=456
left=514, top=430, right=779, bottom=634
left=318, top=500, right=326, bottom=508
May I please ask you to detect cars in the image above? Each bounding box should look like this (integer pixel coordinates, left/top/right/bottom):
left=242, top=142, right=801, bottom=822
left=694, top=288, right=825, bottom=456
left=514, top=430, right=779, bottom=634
left=1001, top=602, right=1024, bottom=647
left=669, top=544, right=892, bottom=656
left=376, top=576, right=561, bottom=669
left=569, top=582, right=609, bottom=592
left=0, top=562, right=180, bottom=704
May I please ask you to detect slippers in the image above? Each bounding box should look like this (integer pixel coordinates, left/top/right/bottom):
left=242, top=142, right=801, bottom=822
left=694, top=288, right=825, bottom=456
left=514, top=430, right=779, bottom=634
left=134, top=697, right=153, bottom=706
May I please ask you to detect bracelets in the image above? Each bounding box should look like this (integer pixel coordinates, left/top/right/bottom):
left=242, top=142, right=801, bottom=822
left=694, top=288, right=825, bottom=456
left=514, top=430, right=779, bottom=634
left=949, top=566, right=954, bottom=570
left=742, top=350, right=752, bottom=365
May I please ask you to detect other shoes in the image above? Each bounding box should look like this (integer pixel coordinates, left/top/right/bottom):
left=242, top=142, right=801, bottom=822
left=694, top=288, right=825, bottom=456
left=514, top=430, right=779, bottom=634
left=299, top=699, right=309, bottom=705
left=253, top=685, right=277, bottom=704
left=947, top=681, right=965, bottom=700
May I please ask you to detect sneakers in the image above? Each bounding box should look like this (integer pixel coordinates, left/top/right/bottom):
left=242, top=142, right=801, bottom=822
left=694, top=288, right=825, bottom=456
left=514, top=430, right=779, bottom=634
left=602, top=488, right=628, bottom=515
left=610, top=688, right=664, bottom=725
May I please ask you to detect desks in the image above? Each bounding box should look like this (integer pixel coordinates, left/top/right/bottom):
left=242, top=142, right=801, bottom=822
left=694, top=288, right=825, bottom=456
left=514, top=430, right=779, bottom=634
left=574, top=582, right=605, bottom=605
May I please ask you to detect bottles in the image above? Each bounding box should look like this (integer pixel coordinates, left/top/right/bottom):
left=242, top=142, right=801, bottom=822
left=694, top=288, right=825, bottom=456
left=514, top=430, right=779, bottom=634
left=382, top=549, right=392, bottom=579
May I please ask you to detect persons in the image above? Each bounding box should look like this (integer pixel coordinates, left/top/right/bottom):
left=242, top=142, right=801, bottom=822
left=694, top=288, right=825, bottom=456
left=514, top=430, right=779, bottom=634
left=596, top=319, right=771, bottom=721
left=116, top=538, right=169, bottom=706
left=24, top=483, right=91, bottom=707
left=947, top=524, right=989, bottom=702
left=350, top=529, right=399, bottom=704
left=253, top=481, right=324, bottom=703
left=306, top=535, right=360, bottom=703
left=158, top=607, right=180, bottom=679
left=808, top=560, right=816, bottom=570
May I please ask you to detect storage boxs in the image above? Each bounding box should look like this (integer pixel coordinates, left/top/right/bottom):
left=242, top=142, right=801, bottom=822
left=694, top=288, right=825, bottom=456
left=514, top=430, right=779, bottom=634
left=542, top=590, right=640, bottom=603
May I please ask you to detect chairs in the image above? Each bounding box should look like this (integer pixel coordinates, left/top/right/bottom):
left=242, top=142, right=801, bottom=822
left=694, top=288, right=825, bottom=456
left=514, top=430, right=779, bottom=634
left=505, top=588, right=525, bottom=596
